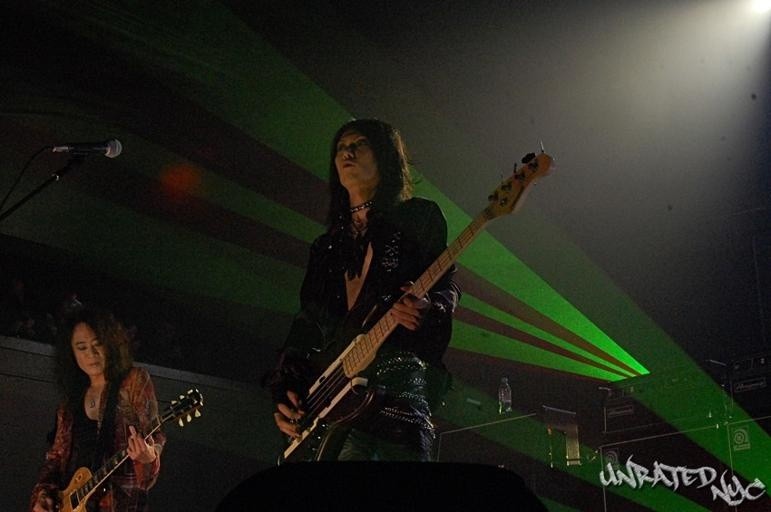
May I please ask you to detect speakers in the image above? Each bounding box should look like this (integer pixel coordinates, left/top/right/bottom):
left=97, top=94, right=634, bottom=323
left=599, top=422, right=726, bottom=512
left=727, top=415, right=771, bottom=512
left=267, top=460, right=547, bottom=512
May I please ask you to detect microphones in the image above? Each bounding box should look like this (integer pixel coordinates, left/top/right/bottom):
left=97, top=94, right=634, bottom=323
left=52, top=138, right=122, bottom=158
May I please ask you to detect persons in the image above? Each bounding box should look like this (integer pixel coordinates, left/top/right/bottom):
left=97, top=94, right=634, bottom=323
left=270, top=118, right=460, bottom=462
left=26, top=308, right=167, bottom=511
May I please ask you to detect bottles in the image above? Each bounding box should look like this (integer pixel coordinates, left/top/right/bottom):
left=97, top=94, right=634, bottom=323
left=498, top=377, right=512, bottom=415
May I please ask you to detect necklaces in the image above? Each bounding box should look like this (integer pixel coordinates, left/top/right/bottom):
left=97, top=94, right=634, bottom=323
left=347, top=199, right=374, bottom=213
left=90, top=400, right=95, bottom=409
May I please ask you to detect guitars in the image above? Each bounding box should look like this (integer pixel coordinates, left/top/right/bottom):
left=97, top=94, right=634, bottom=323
left=35, top=387, right=204, bottom=512
left=273, top=153, right=554, bottom=462
